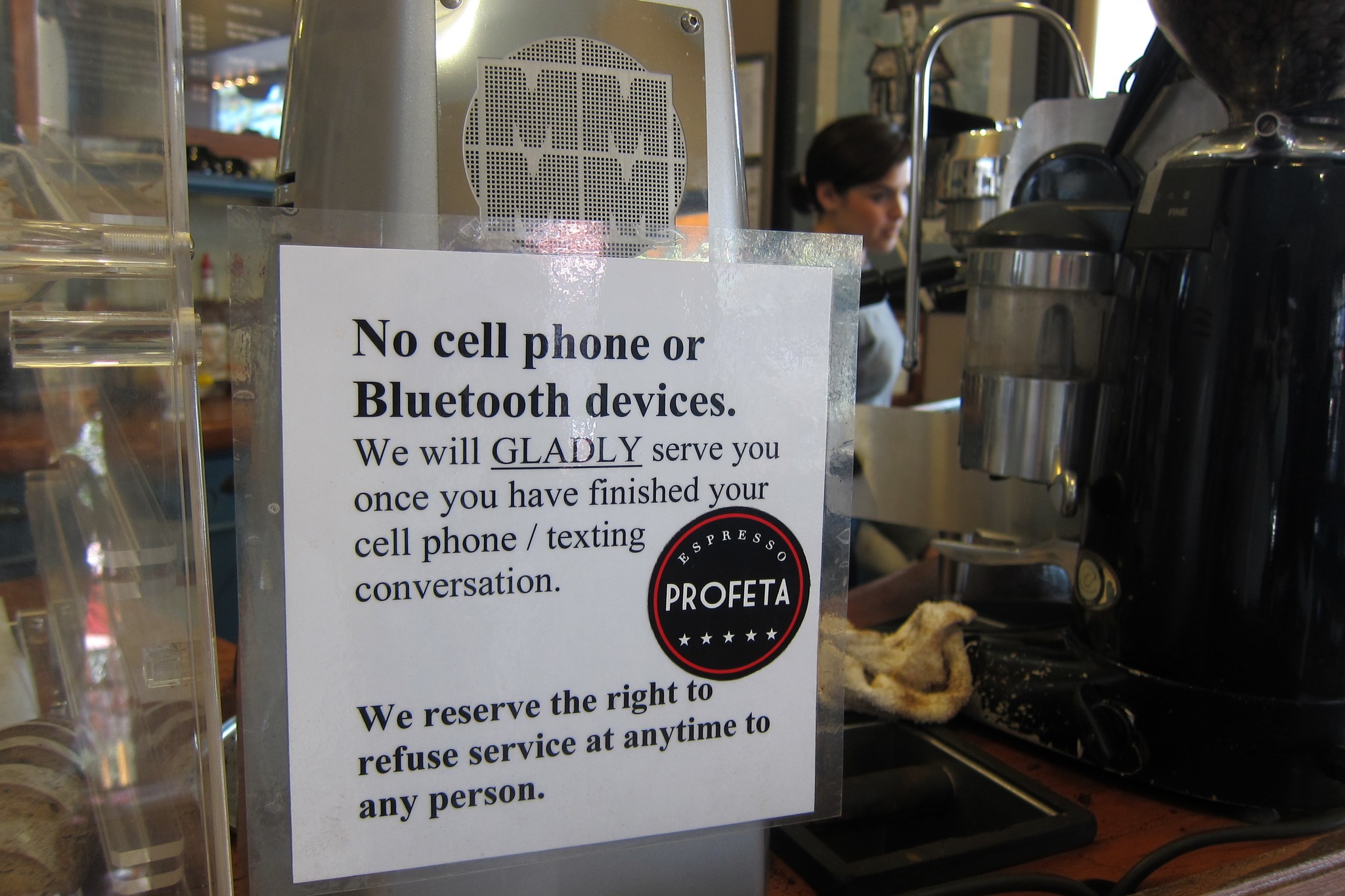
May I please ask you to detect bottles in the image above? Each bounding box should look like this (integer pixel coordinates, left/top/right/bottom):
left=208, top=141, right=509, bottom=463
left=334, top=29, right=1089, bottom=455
left=220, top=255, right=266, bottom=399
left=195, top=255, right=229, bottom=400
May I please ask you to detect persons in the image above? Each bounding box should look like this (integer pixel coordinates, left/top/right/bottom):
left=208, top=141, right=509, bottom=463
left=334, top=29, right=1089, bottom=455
left=746, top=111, right=917, bottom=574
